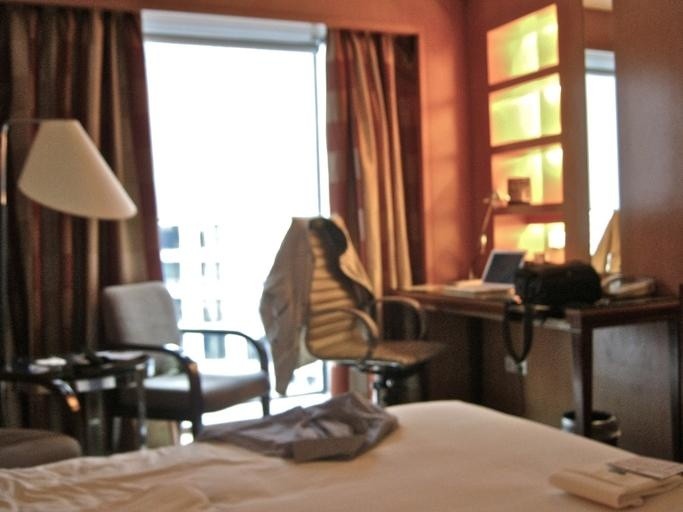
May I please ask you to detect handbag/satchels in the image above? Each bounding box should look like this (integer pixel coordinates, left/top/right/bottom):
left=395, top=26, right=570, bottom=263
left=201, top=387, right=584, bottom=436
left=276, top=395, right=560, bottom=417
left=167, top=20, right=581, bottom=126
left=502, top=262, right=600, bottom=364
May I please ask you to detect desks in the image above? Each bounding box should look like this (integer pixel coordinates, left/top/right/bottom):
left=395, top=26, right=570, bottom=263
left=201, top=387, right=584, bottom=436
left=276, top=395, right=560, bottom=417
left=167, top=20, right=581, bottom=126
left=28, top=349, right=152, bottom=456
left=389, top=281, right=683, bottom=463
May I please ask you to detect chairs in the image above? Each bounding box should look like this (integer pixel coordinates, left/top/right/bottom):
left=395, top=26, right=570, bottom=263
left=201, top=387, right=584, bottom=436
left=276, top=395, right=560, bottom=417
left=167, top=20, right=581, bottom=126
left=100, top=279, right=271, bottom=443
left=291, top=214, right=443, bottom=406
left=0, top=371, right=83, bottom=469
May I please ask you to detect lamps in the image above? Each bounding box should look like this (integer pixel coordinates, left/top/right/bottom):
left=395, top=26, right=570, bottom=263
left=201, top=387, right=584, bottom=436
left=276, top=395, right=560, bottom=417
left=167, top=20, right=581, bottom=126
left=0, top=117, right=138, bottom=371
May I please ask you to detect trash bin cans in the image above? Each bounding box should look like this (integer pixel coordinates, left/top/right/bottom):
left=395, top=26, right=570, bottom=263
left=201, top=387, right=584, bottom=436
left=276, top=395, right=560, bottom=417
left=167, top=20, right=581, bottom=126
left=560, top=410, right=623, bottom=450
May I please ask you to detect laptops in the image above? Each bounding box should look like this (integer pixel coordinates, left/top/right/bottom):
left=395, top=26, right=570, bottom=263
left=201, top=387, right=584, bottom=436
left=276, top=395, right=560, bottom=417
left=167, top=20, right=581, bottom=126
left=441, top=247, right=527, bottom=293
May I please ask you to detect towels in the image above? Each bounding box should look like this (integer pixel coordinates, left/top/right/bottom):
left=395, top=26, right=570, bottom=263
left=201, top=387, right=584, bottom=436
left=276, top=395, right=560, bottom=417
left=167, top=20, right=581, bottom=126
left=551, top=451, right=682, bottom=507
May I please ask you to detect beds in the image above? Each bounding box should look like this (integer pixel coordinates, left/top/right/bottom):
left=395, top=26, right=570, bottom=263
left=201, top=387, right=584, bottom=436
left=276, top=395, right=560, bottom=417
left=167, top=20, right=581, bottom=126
left=1, top=398, right=682, bottom=511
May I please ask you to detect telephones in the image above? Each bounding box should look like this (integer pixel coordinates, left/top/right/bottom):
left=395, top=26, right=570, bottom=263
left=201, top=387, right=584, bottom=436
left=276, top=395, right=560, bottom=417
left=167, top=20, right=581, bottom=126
left=599, top=271, right=657, bottom=300
